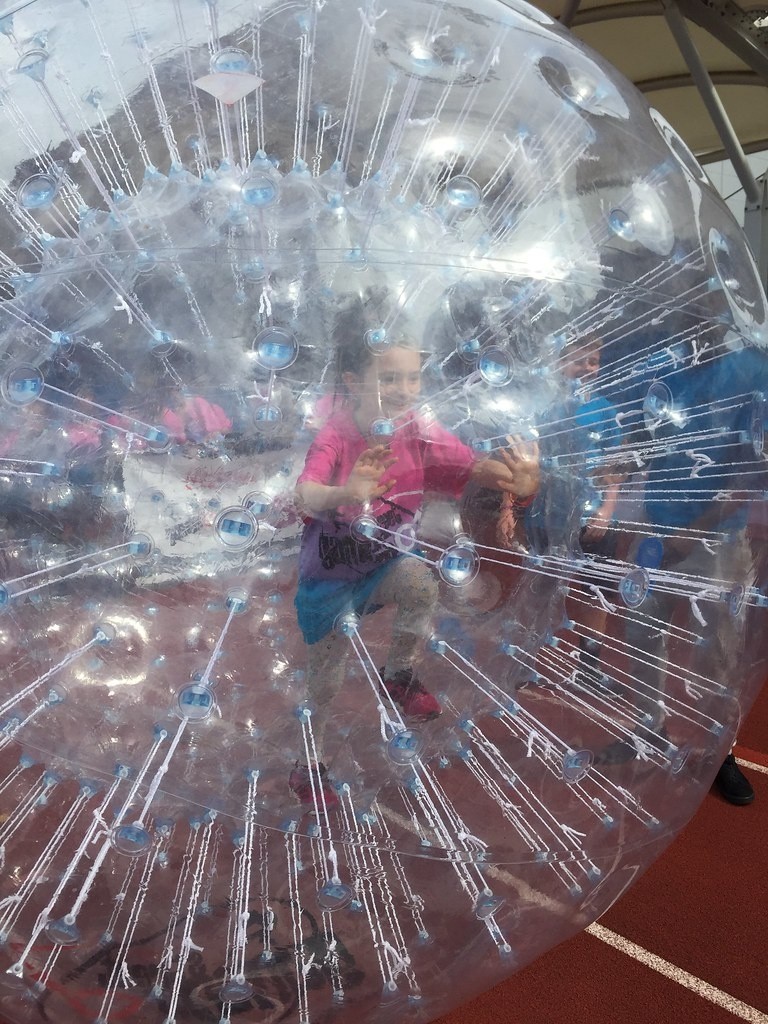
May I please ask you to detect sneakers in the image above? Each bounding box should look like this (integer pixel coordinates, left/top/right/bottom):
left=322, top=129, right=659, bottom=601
left=592, top=728, right=670, bottom=761
left=377, top=664, right=440, bottom=724
left=702, top=755, right=753, bottom=805
left=287, top=762, right=341, bottom=815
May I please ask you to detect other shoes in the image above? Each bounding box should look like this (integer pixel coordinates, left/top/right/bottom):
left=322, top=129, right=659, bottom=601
left=574, top=668, right=628, bottom=700
left=515, top=659, right=536, bottom=686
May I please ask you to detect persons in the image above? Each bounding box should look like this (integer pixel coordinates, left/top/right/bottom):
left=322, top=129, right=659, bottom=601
left=499, top=337, right=635, bottom=695
left=287, top=285, right=541, bottom=810
left=578, top=243, right=766, bottom=804
left=112, top=345, right=231, bottom=454
left=5, top=345, right=109, bottom=451
